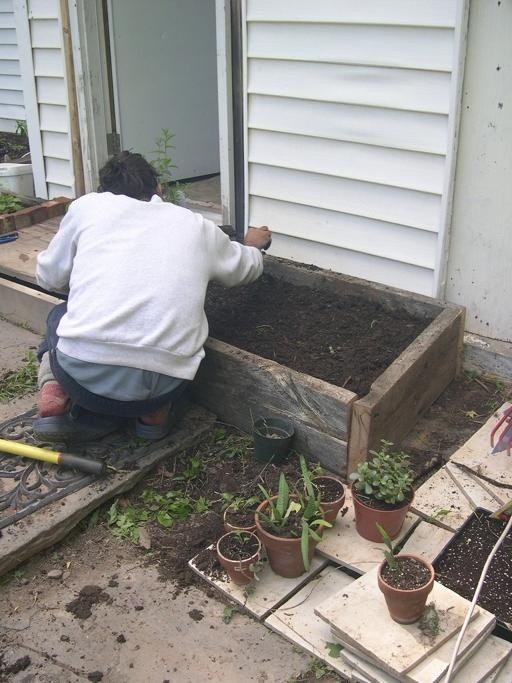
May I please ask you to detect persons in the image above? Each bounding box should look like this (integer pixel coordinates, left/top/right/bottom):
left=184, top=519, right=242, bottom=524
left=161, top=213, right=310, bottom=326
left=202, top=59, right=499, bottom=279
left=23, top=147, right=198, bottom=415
left=35, top=150, right=272, bottom=444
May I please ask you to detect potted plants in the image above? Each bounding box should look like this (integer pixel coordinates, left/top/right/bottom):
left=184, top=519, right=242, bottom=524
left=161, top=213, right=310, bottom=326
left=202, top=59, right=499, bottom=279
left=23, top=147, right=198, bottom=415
left=372, top=521, right=434, bottom=625
left=217, top=529, right=264, bottom=587
left=350, top=438, right=415, bottom=542
left=255, top=453, right=333, bottom=577
left=221, top=494, right=261, bottom=532
left=301, top=461, right=345, bottom=526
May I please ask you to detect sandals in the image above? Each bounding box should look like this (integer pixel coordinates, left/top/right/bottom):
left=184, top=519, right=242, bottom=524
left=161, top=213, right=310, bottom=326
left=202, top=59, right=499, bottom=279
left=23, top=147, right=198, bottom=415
left=32, top=396, right=83, bottom=443
left=133, top=384, right=187, bottom=439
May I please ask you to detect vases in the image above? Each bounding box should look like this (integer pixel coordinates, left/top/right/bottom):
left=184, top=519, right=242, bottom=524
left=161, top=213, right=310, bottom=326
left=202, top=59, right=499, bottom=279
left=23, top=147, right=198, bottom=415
left=253, top=417, right=295, bottom=467
